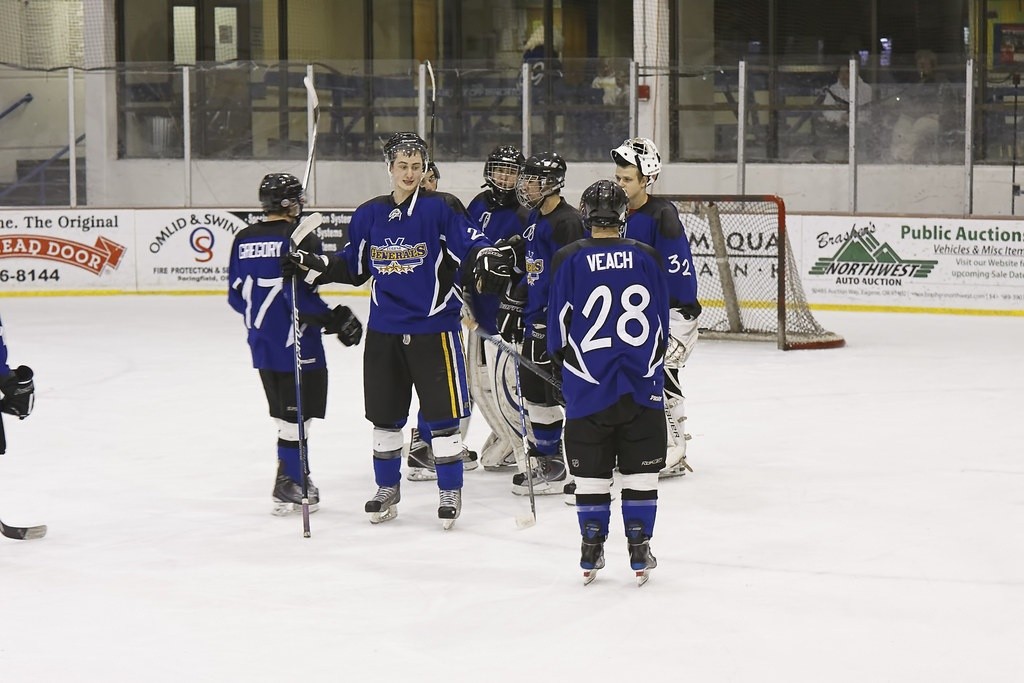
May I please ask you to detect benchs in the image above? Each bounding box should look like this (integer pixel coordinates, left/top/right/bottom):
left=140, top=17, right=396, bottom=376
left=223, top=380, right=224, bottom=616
left=181, top=51, right=1023, bottom=165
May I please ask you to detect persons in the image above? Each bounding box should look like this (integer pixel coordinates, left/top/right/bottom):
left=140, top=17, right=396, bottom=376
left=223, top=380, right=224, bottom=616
left=591, top=56, right=630, bottom=159
left=548, top=180, right=670, bottom=588
left=515, top=26, right=566, bottom=139
left=228, top=173, right=363, bottom=516
left=811, top=49, right=956, bottom=161
left=611, top=138, right=701, bottom=478
left=461, top=145, right=590, bottom=503
left=282, top=131, right=510, bottom=531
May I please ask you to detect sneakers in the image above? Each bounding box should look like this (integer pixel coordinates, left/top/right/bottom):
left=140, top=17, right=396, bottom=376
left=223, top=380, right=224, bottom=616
left=438, top=489, right=462, bottom=530
left=408, top=428, right=477, bottom=480
left=273, top=463, right=320, bottom=517
left=364, top=483, right=400, bottom=524
left=511, top=441, right=575, bottom=495
left=579, top=538, right=606, bottom=585
left=627, top=538, right=657, bottom=585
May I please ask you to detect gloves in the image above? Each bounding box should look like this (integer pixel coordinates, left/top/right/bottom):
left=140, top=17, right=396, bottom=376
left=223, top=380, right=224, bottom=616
left=494, top=235, right=525, bottom=258
left=495, top=295, right=527, bottom=342
left=324, top=304, right=363, bottom=346
left=475, top=247, right=511, bottom=295
left=0, top=366, right=34, bottom=422
left=281, top=250, right=343, bottom=285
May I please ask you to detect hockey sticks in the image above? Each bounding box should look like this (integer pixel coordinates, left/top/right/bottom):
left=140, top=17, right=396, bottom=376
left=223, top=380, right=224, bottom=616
left=288, top=208, right=324, bottom=538
left=473, top=324, right=563, bottom=389
left=0, top=521, right=49, bottom=541
left=302, top=77, right=322, bottom=192
left=664, top=389, right=687, bottom=467
left=510, top=331, right=538, bottom=529
left=425, top=59, right=437, bottom=161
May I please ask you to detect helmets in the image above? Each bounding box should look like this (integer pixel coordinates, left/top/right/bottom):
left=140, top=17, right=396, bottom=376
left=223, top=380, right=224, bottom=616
left=580, top=179, right=629, bottom=232
left=259, top=174, right=304, bottom=213
left=384, top=131, right=430, bottom=162
left=610, top=137, right=662, bottom=187
left=515, top=152, right=565, bottom=210
left=481, top=145, right=525, bottom=193
left=423, top=160, right=441, bottom=180
